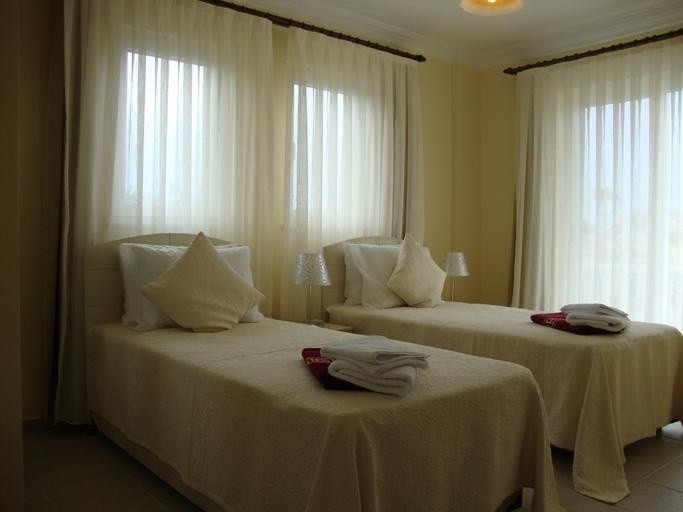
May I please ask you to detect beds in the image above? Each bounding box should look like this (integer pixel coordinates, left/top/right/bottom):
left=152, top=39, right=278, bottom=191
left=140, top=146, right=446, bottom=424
left=327, top=236, right=683, bottom=505
left=83, top=233, right=561, bottom=512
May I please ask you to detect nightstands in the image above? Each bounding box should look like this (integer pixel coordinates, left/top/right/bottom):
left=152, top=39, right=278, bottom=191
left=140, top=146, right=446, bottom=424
left=326, top=323, right=351, bottom=330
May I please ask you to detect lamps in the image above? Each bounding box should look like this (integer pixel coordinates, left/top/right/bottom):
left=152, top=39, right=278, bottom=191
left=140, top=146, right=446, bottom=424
left=461, top=0, right=522, bottom=16
left=290, top=251, right=332, bottom=326
left=441, top=250, right=470, bottom=302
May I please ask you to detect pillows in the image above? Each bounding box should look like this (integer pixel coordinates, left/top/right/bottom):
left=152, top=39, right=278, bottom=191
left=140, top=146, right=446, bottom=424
left=342, top=236, right=446, bottom=313
left=118, top=233, right=264, bottom=327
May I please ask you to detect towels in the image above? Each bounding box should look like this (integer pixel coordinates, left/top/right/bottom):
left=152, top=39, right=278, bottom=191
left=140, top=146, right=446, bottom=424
left=301, top=336, right=429, bottom=396
left=529, top=299, right=629, bottom=337
